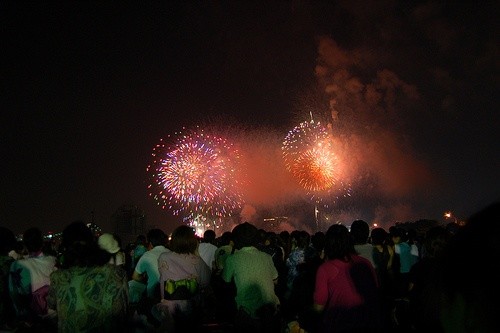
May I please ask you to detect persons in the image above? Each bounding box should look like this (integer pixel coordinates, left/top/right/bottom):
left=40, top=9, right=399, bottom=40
left=0, top=202, right=500, bottom=333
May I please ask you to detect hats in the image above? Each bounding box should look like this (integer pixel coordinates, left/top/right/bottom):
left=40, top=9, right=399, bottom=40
left=98, top=233, right=120, bottom=254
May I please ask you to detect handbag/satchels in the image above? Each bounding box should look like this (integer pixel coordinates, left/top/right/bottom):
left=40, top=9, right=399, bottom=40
left=163, top=276, right=198, bottom=301
